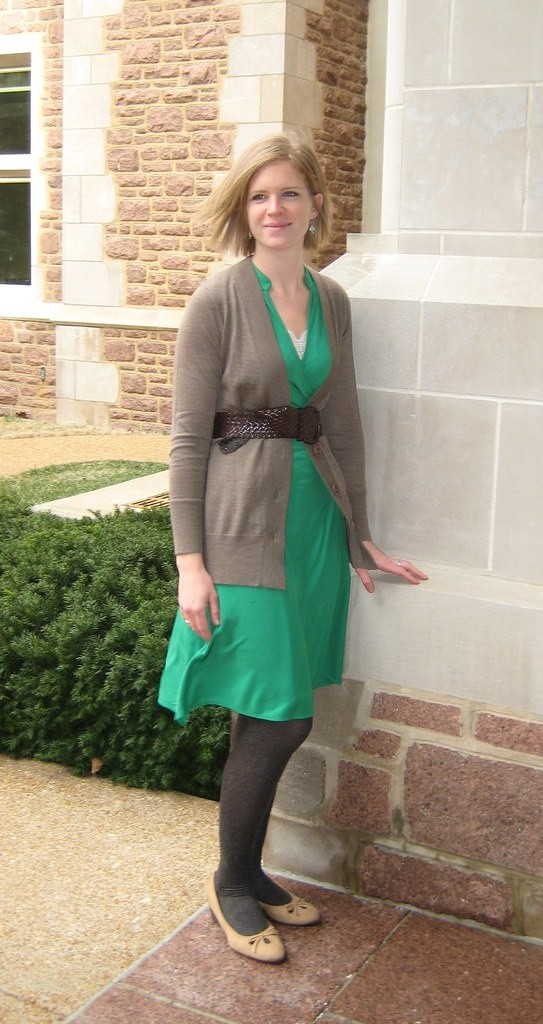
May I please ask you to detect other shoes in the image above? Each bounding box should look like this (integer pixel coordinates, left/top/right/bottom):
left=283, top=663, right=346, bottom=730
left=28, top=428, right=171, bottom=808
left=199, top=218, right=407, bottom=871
left=206, top=871, right=286, bottom=963
left=256, top=869, right=320, bottom=926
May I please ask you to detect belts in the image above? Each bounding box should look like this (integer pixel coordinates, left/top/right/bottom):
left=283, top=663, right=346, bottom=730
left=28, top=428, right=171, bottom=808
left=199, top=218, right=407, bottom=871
left=212, top=406, right=323, bottom=454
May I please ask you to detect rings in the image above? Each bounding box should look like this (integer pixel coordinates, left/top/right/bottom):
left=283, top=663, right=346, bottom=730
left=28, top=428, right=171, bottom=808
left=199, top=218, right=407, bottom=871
left=185, top=620, right=190, bottom=624
left=398, top=559, right=402, bottom=566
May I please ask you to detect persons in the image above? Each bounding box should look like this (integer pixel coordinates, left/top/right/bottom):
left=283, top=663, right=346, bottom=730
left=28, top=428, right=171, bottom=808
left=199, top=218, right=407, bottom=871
left=157, top=136, right=429, bottom=963
